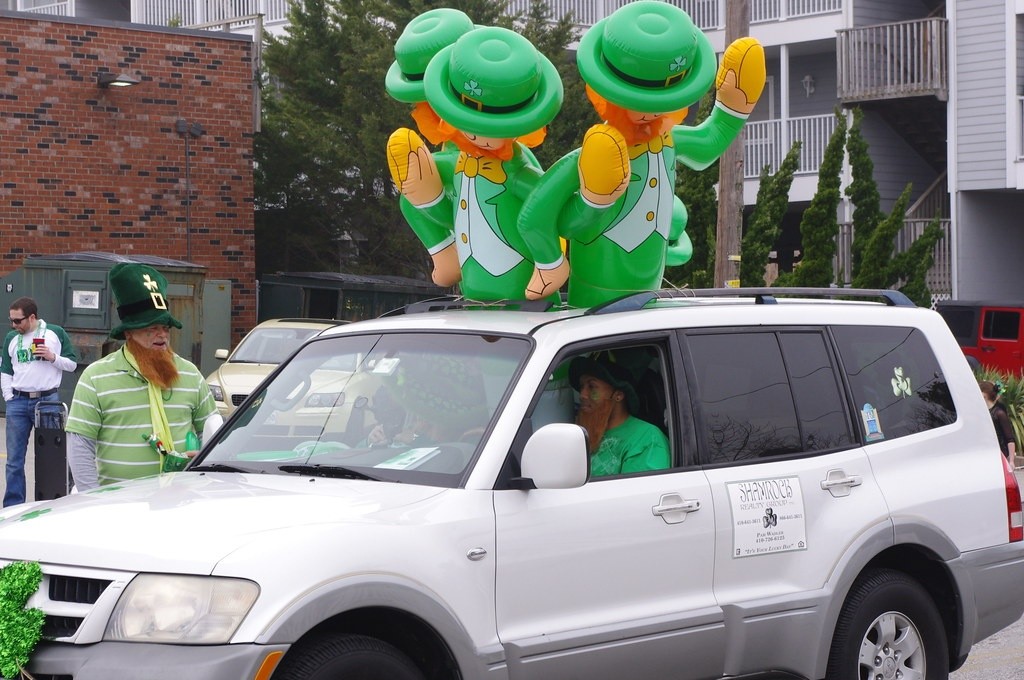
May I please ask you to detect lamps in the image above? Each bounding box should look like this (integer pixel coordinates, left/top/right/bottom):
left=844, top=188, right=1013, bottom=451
left=801, top=75, right=815, bottom=98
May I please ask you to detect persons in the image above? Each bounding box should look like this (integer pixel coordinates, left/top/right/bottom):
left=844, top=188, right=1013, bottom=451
left=64, top=263, right=220, bottom=493
left=979, top=381, right=1015, bottom=471
left=568, top=348, right=673, bottom=477
left=0, top=296, right=77, bottom=507
left=357, top=411, right=451, bottom=450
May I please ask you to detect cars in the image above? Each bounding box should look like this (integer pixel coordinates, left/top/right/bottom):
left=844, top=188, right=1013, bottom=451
left=205, top=318, right=396, bottom=457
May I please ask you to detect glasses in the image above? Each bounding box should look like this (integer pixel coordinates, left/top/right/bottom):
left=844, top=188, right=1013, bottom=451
left=8, top=314, right=30, bottom=324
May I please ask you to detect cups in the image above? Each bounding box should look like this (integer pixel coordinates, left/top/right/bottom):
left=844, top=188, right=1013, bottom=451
left=34, top=338, right=44, bottom=354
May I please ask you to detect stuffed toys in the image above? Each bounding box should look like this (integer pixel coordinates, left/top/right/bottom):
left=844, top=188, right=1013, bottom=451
left=142, top=431, right=166, bottom=455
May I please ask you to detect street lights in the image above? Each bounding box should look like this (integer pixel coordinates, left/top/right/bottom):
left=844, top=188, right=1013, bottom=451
left=175, top=119, right=207, bottom=263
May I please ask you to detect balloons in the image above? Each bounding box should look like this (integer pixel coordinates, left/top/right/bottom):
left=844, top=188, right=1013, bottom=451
left=386, top=1, right=767, bottom=305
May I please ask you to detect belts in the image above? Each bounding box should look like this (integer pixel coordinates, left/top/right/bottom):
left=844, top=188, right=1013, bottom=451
left=13, top=387, right=59, bottom=399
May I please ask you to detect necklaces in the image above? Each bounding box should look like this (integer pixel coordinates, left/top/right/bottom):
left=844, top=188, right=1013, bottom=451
left=17, top=326, right=40, bottom=362
left=122, top=346, right=172, bottom=401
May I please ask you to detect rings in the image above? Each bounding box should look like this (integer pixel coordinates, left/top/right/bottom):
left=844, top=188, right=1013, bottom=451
left=42, top=345, right=44, bottom=347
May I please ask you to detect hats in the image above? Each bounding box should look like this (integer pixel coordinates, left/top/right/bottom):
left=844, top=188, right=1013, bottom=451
left=107, top=260, right=183, bottom=338
left=571, top=351, right=648, bottom=411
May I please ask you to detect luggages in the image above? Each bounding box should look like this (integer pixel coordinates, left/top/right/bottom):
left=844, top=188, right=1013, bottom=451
left=33, top=400, right=73, bottom=501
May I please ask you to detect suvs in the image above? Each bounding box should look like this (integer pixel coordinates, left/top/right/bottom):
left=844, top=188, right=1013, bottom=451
left=1, top=286, right=1023, bottom=678
left=934, top=298, right=1024, bottom=385
